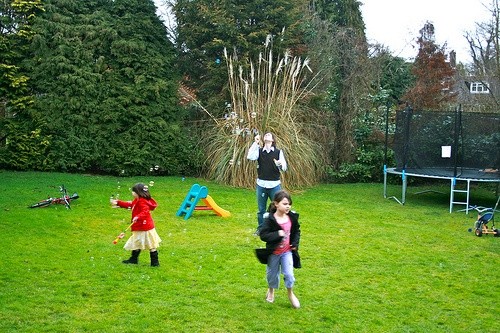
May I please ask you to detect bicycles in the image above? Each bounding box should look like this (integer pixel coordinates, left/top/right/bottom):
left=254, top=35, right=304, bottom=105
left=26, top=183, right=80, bottom=210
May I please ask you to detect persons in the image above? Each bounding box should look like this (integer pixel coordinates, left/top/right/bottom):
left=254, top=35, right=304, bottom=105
left=255, top=191, right=302, bottom=309
left=247, top=132, right=286, bottom=236
left=110, top=183, right=162, bottom=266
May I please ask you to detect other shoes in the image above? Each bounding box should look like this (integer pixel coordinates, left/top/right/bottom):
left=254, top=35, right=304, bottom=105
left=266, top=290, right=274, bottom=302
left=289, top=294, right=300, bottom=308
left=255, top=230, right=260, bottom=236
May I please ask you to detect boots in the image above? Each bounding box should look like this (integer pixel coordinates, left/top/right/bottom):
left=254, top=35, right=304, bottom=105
left=123, top=250, right=140, bottom=264
left=150, top=251, right=159, bottom=266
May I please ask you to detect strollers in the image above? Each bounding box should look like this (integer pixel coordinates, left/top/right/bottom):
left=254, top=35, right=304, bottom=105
left=468, top=196, right=500, bottom=238
